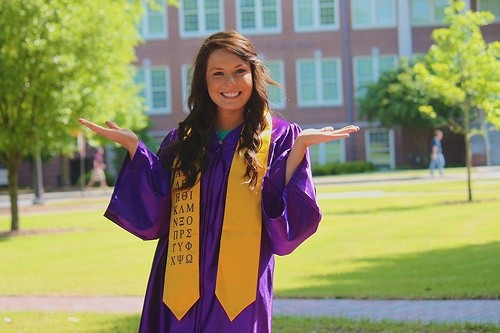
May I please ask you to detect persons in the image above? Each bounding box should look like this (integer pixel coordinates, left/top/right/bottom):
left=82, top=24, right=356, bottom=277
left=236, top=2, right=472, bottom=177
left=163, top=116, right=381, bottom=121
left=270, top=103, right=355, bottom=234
left=77, top=31, right=360, bottom=333
left=430, top=130, right=445, bottom=178
left=86, top=147, right=108, bottom=189
